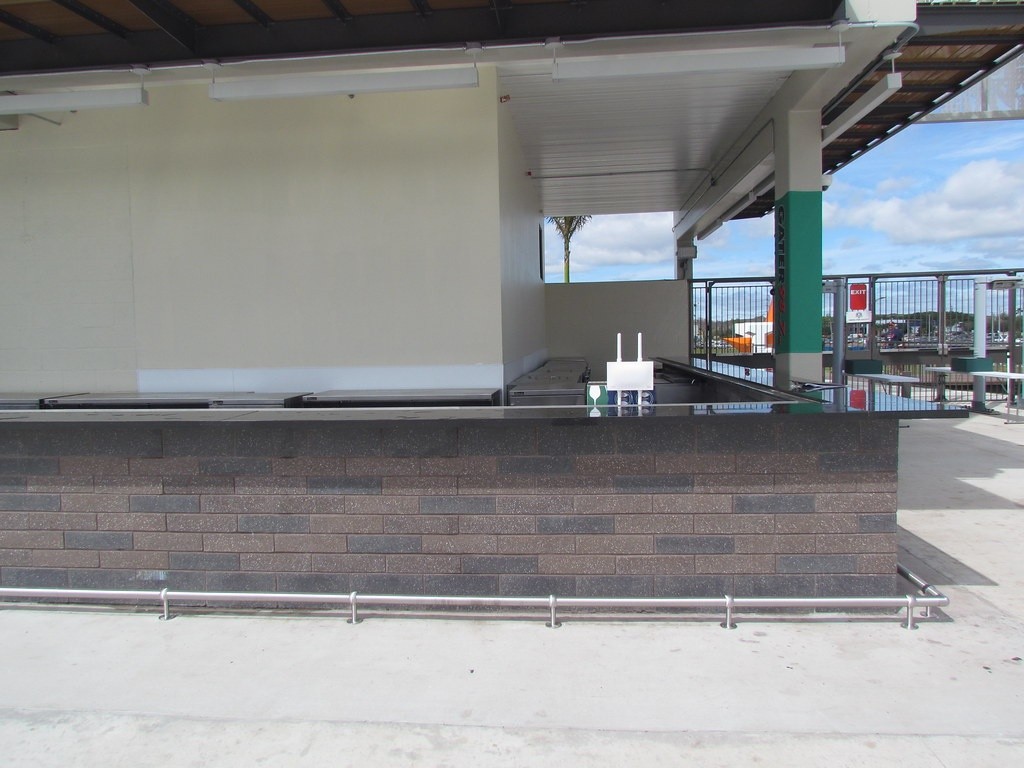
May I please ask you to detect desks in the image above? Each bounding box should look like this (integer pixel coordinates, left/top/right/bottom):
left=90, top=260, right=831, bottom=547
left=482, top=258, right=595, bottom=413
left=841, top=370, right=920, bottom=395
left=925, top=366, right=952, bottom=402
left=969, top=371, right=1024, bottom=425
